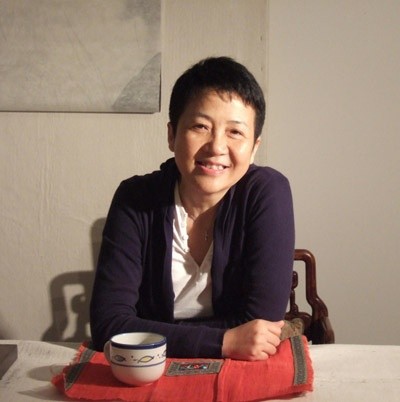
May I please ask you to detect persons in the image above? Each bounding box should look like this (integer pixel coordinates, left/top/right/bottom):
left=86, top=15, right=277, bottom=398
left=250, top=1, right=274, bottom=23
left=89, top=56, right=296, bottom=362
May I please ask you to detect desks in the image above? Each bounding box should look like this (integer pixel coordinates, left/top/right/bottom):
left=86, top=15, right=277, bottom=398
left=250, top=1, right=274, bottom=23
left=0, top=339, right=400, bottom=402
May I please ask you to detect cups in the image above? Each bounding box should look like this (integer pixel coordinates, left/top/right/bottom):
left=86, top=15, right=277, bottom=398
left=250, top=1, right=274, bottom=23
left=104, top=332, right=167, bottom=386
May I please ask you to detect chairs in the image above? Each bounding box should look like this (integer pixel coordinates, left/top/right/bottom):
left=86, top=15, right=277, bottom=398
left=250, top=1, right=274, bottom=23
left=286, top=249, right=334, bottom=344
